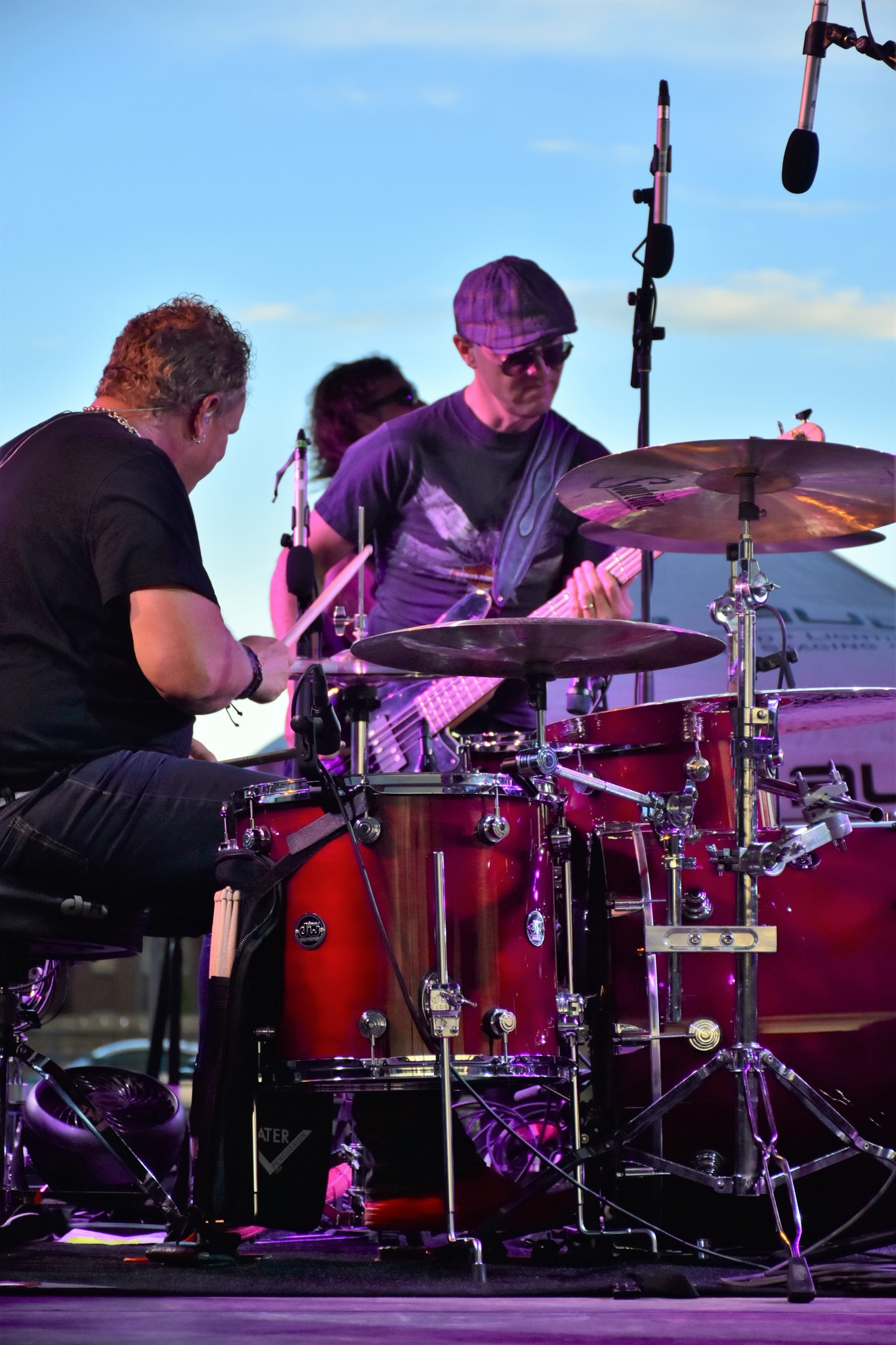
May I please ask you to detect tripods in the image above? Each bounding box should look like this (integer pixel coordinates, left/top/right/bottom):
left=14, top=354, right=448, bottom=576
left=579, top=487, right=895, bottom=1303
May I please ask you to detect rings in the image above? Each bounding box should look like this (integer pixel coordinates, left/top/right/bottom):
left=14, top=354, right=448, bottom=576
left=581, top=603, right=595, bottom=611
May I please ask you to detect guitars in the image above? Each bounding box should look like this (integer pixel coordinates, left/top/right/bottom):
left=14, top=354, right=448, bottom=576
left=283, top=407, right=824, bottom=778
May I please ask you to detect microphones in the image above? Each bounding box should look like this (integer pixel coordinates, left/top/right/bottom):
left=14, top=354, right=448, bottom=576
left=287, top=429, right=313, bottom=596
left=779, top=0, right=830, bottom=194
left=647, top=77, right=674, bottom=279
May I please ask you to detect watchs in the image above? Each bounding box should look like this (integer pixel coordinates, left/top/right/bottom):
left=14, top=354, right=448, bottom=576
left=233, top=645, right=263, bottom=701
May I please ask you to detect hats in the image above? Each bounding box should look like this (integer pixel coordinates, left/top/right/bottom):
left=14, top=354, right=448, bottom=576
left=453, top=256, right=576, bottom=353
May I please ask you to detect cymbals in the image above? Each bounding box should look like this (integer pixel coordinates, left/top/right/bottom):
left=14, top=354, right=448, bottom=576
left=554, top=438, right=896, bottom=544
left=349, top=617, right=727, bottom=680
left=533, top=686, right=896, bottom=745
left=577, top=519, right=887, bottom=555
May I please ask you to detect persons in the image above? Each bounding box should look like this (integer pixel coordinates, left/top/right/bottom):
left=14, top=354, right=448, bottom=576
left=0, top=297, right=492, bottom=1200
left=269, top=255, right=634, bottom=780
left=322, top=354, right=431, bottom=670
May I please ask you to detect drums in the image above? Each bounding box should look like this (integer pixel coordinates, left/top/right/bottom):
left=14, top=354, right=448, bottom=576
left=220, top=771, right=575, bottom=1100
left=575, top=821, right=896, bottom=1154
left=531, top=736, right=776, bottom=966
left=453, top=730, right=537, bottom=781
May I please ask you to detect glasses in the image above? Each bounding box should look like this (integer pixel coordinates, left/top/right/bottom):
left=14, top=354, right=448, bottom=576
left=479, top=334, right=574, bottom=378
left=363, top=383, right=417, bottom=414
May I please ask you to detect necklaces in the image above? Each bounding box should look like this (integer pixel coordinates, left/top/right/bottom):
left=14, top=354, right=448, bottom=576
left=81, top=405, right=146, bottom=443
